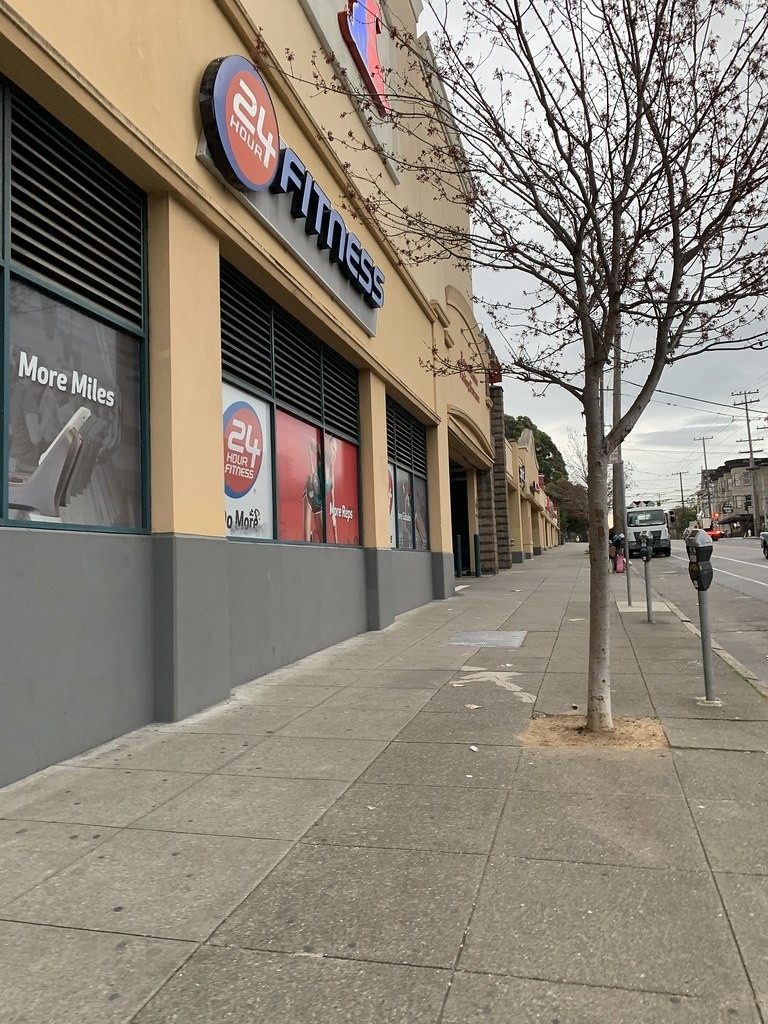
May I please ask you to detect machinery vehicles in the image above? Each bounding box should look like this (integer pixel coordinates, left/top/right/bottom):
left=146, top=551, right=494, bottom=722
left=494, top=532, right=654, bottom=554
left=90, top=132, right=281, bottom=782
left=683, top=516, right=720, bottom=542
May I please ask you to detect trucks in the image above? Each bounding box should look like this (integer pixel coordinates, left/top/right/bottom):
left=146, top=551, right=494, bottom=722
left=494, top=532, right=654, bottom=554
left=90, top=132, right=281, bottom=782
left=624, top=500, right=671, bottom=560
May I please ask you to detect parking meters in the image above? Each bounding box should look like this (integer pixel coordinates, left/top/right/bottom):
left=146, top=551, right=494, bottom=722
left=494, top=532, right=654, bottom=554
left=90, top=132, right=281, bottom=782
left=638, top=529, right=654, bottom=624
left=686, top=529, right=716, bottom=701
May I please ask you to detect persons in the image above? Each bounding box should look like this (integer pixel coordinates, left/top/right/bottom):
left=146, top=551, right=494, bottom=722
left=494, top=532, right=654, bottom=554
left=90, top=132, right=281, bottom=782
left=301, top=435, right=335, bottom=543
left=9, top=343, right=83, bottom=473
left=402, top=480, right=427, bottom=549
